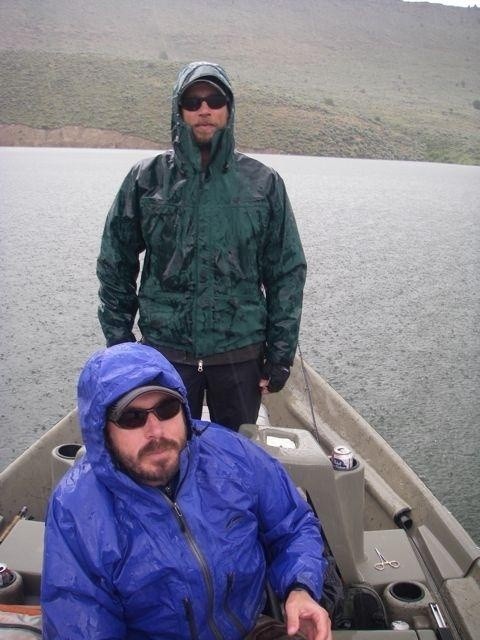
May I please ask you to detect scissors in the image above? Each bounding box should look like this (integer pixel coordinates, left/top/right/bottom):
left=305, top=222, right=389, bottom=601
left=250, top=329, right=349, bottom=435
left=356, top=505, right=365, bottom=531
left=373, top=547, right=400, bottom=570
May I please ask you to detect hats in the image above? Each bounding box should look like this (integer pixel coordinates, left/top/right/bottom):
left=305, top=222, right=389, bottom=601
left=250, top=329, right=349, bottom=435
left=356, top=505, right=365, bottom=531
left=177, top=76, right=231, bottom=106
left=106, top=384, right=186, bottom=423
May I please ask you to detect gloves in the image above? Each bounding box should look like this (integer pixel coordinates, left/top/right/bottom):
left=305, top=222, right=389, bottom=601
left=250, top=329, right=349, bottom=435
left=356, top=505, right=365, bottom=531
left=261, top=361, right=292, bottom=394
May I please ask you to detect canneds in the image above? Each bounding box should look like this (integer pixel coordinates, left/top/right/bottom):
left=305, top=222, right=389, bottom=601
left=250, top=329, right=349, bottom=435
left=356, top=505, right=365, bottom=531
left=331, top=446, right=354, bottom=471
left=0, top=563, right=12, bottom=587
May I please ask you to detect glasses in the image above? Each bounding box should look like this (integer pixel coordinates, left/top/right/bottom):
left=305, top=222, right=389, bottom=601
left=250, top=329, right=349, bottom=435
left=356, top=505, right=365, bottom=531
left=107, top=395, right=181, bottom=430
left=178, top=94, right=229, bottom=111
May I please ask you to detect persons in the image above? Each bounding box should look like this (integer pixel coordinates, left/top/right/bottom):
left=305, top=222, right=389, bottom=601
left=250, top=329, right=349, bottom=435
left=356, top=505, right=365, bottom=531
left=40, top=341, right=335, bottom=640
left=94, top=60, right=308, bottom=431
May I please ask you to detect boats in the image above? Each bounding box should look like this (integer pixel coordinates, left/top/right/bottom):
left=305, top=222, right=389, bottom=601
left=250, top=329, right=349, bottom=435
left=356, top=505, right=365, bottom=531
left=2, top=344, right=480, bottom=639
